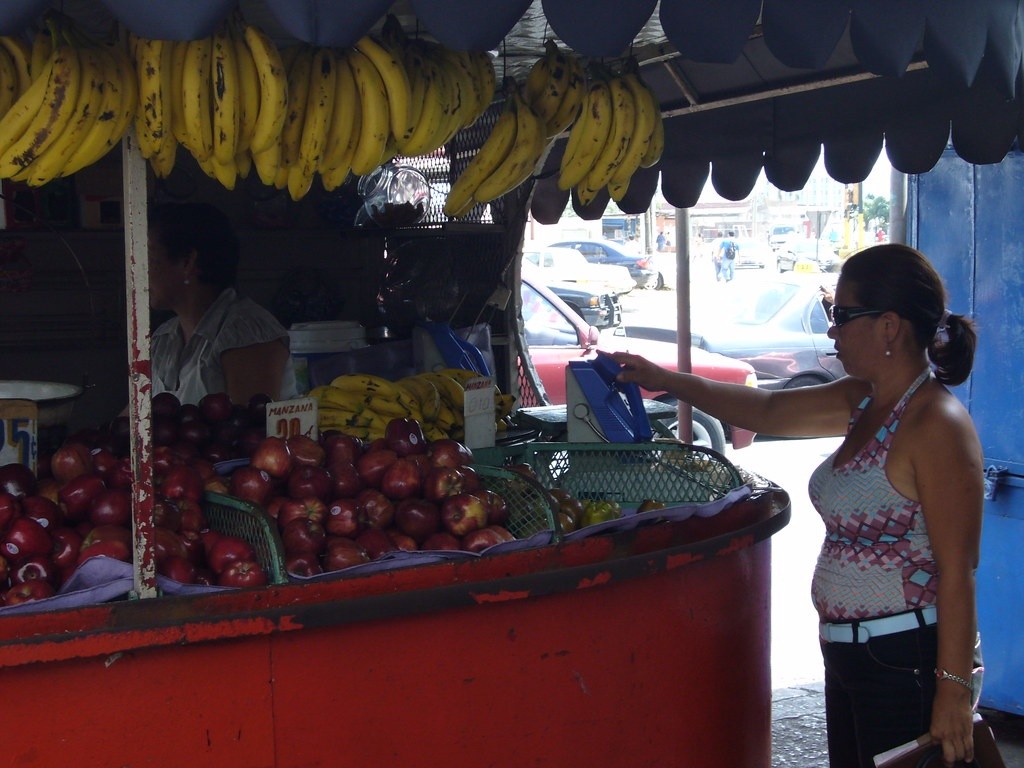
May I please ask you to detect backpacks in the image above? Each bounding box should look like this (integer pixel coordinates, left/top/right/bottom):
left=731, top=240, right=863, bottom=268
left=727, top=241, right=736, bottom=260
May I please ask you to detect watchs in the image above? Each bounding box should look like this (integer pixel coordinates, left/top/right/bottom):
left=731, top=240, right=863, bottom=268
left=934, top=668, right=973, bottom=691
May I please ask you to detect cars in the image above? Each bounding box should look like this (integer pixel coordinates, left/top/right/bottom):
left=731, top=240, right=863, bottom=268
left=507, top=227, right=677, bottom=332
left=516, top=273, right=761, bottom=460
left=698, top=275, right=851, bottom=391
left=777, top=235, right=837, bottom=273
left=767, top=223, right=799, bottom=250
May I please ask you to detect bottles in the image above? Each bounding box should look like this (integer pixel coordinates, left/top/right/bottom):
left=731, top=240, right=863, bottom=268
left=357, top=162, right=431, bottom=227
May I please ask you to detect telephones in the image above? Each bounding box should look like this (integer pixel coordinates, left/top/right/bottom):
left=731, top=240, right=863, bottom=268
left=412, top=319, right=491, bottom=378
left=564, top=353, right=654, bottom=443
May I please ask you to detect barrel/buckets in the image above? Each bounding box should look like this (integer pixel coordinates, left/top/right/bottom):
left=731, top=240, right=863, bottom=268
left=287, top=319, right=372, bottom=399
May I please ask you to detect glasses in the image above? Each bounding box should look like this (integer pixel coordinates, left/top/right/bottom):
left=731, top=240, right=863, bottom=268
left=830, top=305, right=887, bottom=328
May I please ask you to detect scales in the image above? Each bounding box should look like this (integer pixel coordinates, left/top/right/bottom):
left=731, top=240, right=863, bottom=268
left=0, top=379, right=86, bottom=457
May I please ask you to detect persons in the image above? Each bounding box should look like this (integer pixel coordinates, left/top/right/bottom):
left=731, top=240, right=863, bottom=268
left=829, top=227, right=886, bottom=246
left=602, top=230, right=742, bottom=283
left=116, top=199, right=298, bottom=405
left=597, top=243, right=986, bottom=768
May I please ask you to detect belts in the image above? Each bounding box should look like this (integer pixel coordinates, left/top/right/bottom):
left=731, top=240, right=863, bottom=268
left=818, top=607, right=938, bottom=644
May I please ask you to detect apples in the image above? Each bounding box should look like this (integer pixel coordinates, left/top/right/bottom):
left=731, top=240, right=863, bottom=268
left=0, top=392, right=519, bottom=608
left=495, top=463, right=665, bottom=535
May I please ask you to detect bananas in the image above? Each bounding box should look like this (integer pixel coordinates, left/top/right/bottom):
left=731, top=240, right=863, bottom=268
left=0, top=13, right=665, bottom=215
left=308, top=370, right=516, bottom=443
